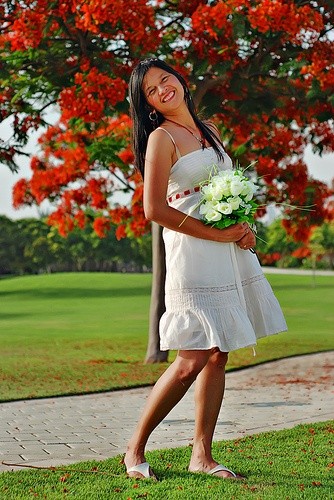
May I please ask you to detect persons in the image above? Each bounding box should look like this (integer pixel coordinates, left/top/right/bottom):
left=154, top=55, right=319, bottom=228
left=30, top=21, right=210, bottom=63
left=122, top=58, right=287, bottom=481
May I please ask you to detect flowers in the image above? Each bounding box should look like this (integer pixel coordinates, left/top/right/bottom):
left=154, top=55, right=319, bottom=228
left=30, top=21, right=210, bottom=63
left=177, top=159, right=318, bottom=257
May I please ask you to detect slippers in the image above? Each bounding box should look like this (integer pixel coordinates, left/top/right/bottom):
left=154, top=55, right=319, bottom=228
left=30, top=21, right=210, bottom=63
left=207, top=464, right=237, bottom=481
left=126, top=462, right=159, bottom=484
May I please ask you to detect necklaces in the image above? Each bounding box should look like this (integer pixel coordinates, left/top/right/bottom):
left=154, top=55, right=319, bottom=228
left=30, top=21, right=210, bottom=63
left=163, top=118, right=206, bottom=150
